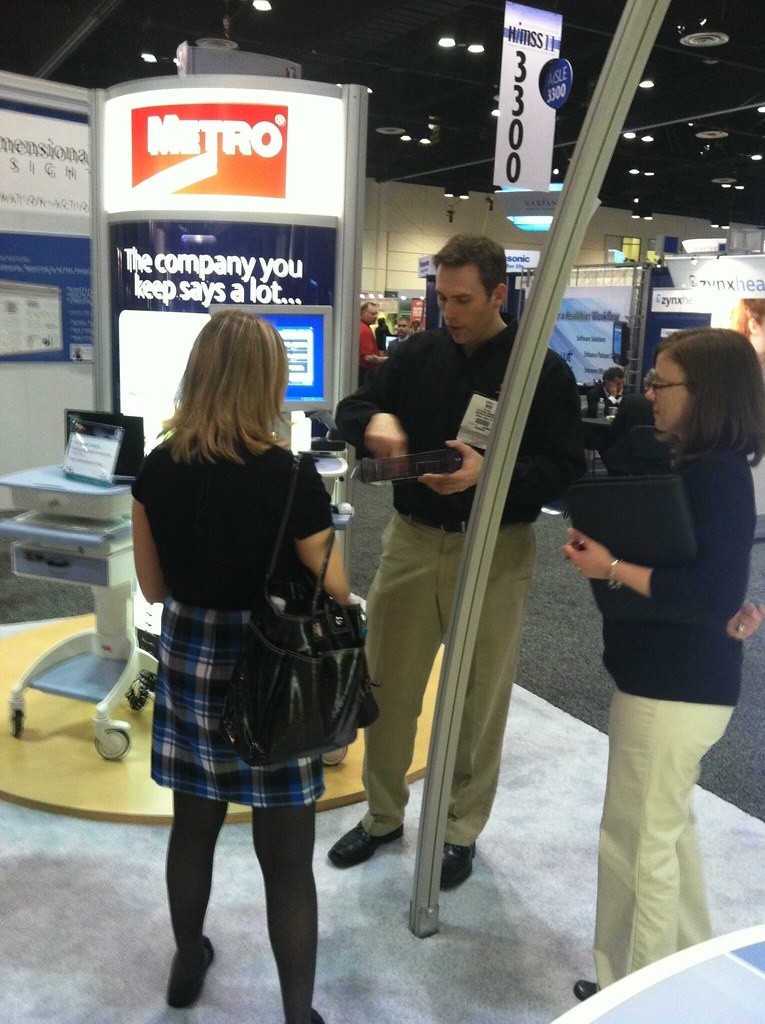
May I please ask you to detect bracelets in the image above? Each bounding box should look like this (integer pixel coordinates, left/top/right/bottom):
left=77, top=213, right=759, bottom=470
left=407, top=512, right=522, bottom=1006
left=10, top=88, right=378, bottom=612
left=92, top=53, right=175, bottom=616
left=608, top=559, right=622, bottom=590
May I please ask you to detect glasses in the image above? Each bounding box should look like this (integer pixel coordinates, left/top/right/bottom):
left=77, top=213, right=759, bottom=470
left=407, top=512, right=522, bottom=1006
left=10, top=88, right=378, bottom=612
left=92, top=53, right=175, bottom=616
left=643, top=368, right=688, bottom=395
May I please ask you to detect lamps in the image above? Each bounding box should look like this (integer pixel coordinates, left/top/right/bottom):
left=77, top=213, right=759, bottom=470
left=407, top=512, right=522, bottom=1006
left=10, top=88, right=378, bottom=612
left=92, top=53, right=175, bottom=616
left=711, top=214, right=730, bottom=230
left=631, top=203, right=651, bottom=220
left=444, top=182, right=470, bottom=201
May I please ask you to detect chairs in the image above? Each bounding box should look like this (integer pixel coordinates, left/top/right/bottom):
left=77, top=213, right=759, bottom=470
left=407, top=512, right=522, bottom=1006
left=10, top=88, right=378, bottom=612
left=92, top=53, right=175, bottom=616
left=622, top=425, right=663, bottom=474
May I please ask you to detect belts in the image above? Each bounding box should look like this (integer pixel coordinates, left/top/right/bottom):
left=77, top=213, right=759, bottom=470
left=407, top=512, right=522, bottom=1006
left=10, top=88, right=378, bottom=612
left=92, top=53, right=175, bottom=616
left=410, top=516, right=471, bottom=535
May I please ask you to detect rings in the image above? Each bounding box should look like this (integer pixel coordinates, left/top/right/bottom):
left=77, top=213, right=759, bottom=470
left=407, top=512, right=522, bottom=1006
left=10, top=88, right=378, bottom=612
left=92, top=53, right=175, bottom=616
left=734, top=624, right=745, bottom=632
left=448, top=490, right=450, bottom=494
left=576, top=567, right=581, bottom=572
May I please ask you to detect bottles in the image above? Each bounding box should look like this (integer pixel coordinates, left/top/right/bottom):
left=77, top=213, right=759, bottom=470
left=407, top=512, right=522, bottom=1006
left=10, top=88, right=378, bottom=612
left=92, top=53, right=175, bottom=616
left=596, top=398, right=606, bottom=419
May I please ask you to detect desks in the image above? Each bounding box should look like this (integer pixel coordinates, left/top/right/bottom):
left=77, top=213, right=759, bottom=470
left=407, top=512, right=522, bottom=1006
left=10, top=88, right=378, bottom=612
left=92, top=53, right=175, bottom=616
left=580, top=415, right=612, bottom=479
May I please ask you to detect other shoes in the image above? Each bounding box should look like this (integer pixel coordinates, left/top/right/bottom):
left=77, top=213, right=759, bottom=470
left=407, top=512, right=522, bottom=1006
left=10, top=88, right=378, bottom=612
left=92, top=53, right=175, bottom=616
left=165, top=935, right=213, bottom=1008
left=574, top=979, right=600, bottom=1001
left=310, top=1007, right=323, bottom=1024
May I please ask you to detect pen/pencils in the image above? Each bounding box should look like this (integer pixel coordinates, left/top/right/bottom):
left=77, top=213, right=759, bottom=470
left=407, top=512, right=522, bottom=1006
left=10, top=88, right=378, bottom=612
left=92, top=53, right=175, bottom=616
left=565, top=539, right=585, bottom=560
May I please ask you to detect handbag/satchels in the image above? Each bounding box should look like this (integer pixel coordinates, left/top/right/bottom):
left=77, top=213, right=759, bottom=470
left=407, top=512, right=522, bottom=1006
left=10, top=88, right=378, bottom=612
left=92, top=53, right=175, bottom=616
left=221, top=453, right=382, bottom=768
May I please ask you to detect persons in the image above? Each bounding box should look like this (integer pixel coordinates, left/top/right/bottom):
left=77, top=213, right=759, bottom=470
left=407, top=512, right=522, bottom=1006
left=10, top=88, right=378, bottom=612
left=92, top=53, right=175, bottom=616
left=334, top=232, right=586, bottom=891
left=375, top=316, right=419, bottom=356
left=130, top=309, right=353, bottom=1024
left=728, top=298, right=765, bottom=360
left=561, top=329, right=765, bottom=1001
left=587, top=365, right=626, bottom=418
left=357, top=302, right=390, bottom=391
left=597, top=368, right=655, bottom=477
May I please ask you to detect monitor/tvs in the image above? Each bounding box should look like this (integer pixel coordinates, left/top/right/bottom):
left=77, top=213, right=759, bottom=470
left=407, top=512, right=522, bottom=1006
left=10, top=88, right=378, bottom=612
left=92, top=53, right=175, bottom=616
left=210, top=303, right=334, bottom=411
left=383, top=334, right=399, bottom=351
left=612, top=320, right=631, bottom=366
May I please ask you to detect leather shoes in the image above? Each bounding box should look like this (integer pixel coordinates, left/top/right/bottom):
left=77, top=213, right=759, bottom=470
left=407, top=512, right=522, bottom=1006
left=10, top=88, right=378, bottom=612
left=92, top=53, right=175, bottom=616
left=439, top=840, right=476, bottom=891
left=326, top=819, right=404, bottom=868
